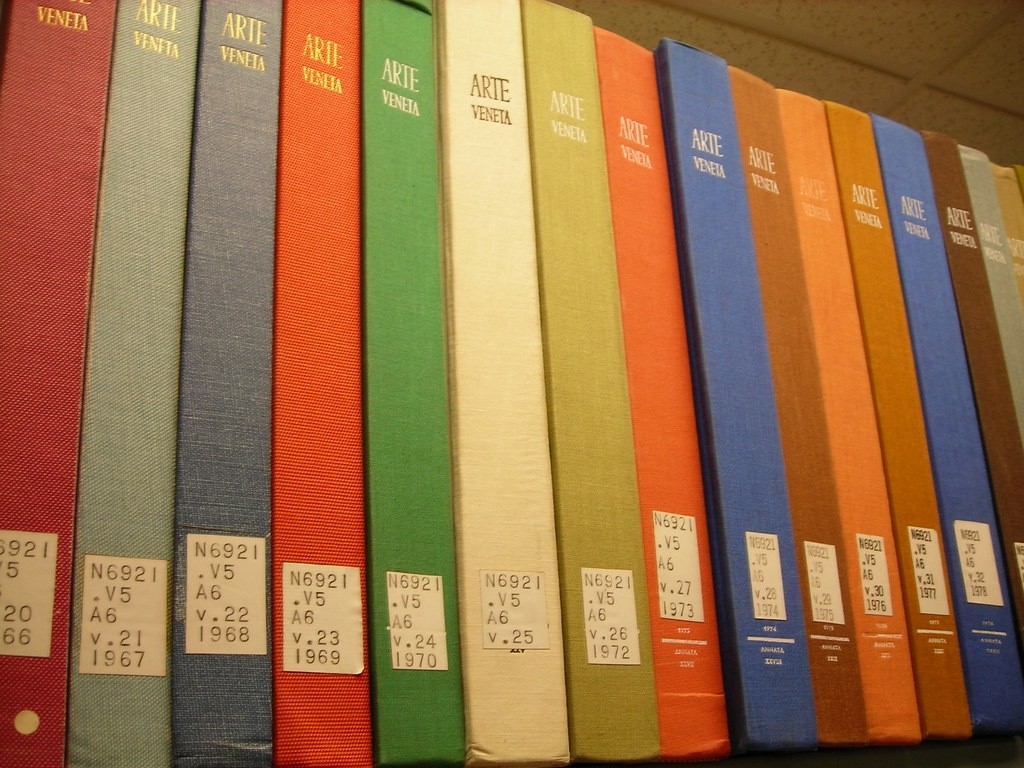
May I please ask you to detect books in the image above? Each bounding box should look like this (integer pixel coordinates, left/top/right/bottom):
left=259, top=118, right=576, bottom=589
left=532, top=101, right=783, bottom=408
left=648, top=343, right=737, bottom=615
left=0, top=0, right=1024, bottom=768
left=649, top=38, right=817, bottom=750
